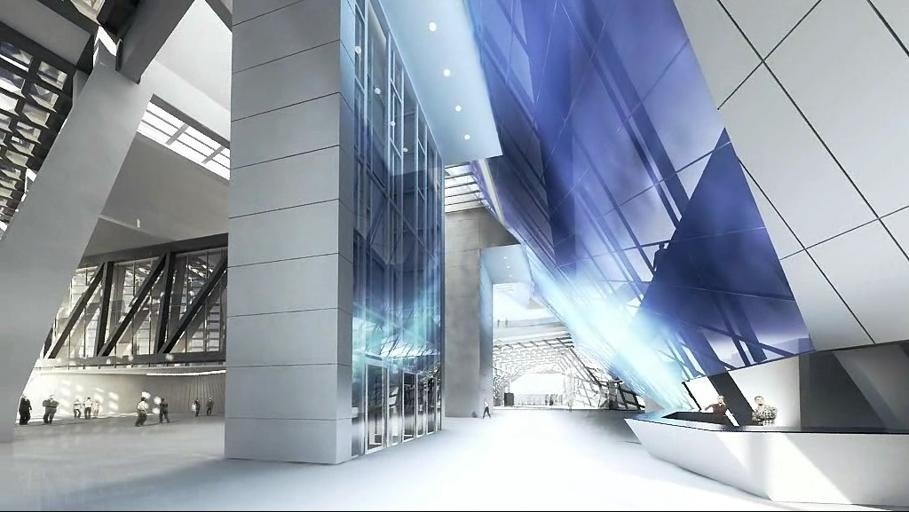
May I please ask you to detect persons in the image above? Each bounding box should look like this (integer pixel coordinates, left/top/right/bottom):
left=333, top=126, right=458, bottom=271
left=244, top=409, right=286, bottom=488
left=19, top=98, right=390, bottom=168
left=19, top=395, right=32, bottom=425
left=705, top=394, right=727, bottom=424
left=191, top=399, right=201, bottom=417
left=135, top=397, right=149, bottom=427
left=482, top=401, right=491, bottom=418
left=42, top=394, right=58, bottom=424
left=159, top=398, right=170, bottom=423
left=93, top=400, right=99, bottom=416
left=206, top=395, right=213, bottom=415
left=73, top=399, right=82, bottom=418
left=84, top=397, right=93, bottom=420
left=750, top=395, right=778, bottom=425
